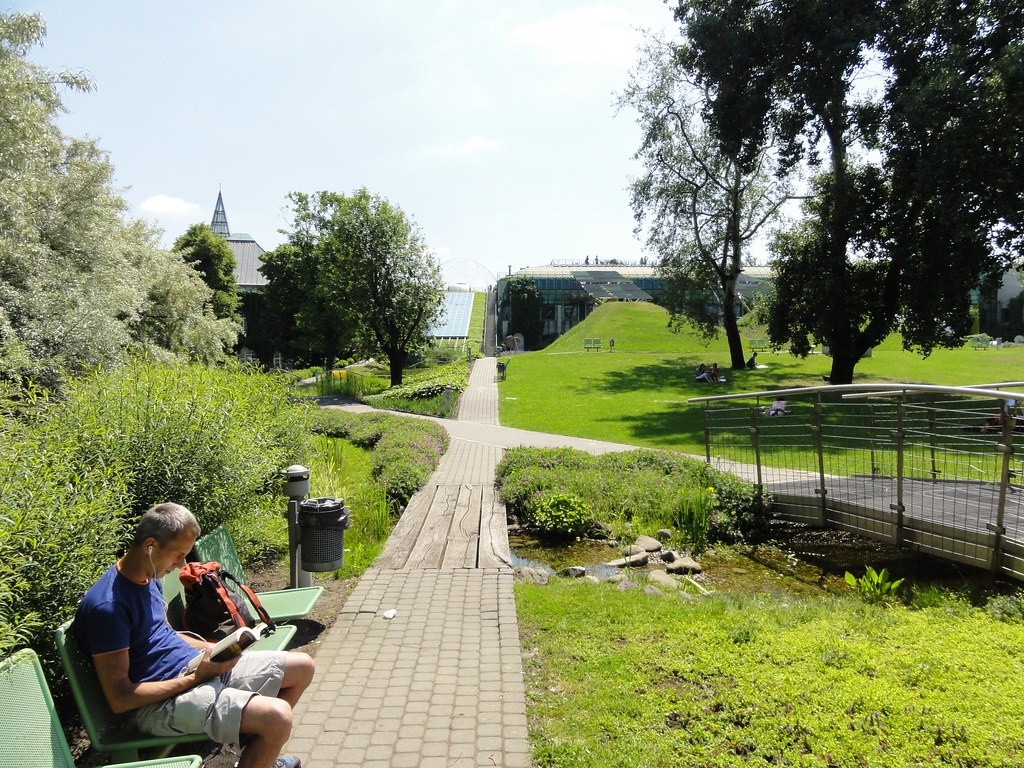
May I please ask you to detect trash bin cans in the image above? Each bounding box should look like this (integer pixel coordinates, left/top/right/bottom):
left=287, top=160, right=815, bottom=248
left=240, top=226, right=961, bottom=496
left=497, top=362, right=504, bottom=374
left=299, top=498, right=349, bottom=573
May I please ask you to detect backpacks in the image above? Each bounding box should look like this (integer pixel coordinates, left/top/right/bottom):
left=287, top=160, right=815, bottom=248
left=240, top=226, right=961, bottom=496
left=179, top=561, right=276, bottom=641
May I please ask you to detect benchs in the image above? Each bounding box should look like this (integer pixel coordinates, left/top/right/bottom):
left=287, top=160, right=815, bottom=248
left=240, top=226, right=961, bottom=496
left=749, top=340, right=767, bottom=352
left=971, top=338, right=989, bottom=350
left=584, top=338, right=601, bottom=352
left=0, top=524, right=323, bottom=768
left=761, top=401, right=790, bottom=417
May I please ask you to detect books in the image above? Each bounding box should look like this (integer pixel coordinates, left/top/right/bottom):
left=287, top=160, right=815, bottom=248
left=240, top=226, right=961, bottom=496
left=188, top=622, right=268, bottom=672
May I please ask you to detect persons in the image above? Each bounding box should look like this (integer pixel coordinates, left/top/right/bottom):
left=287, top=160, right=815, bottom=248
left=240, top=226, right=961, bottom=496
left=74, top=502, right=314, bottom=768
left=696, top=363, right=714, bottom=383
left=770, top=396, right=787, bottom=416
left=707, top=363, right=720, bottom=383
left=745, top=352, right=758, bottom=370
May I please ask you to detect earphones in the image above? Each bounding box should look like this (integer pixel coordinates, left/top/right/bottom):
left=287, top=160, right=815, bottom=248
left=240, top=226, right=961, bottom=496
left=148, top=545, right=154, bottom=555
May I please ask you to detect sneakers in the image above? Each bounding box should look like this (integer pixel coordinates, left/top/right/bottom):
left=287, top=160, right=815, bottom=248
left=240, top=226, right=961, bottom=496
left=233, top=756, right=301, bottom=768
